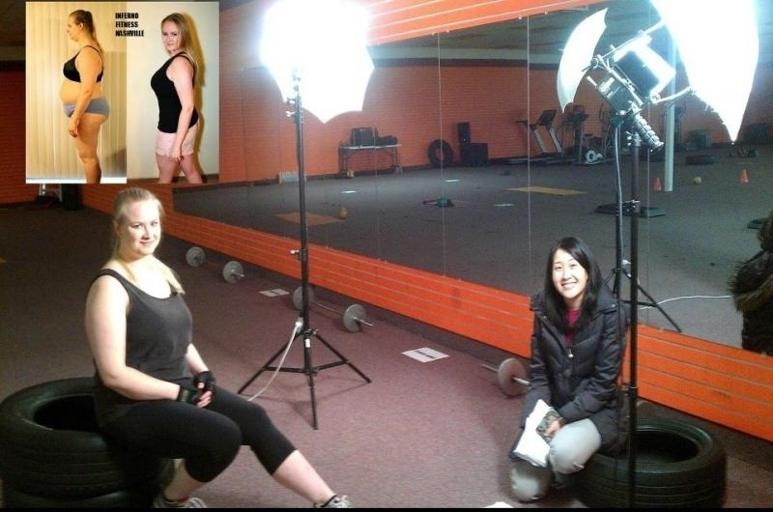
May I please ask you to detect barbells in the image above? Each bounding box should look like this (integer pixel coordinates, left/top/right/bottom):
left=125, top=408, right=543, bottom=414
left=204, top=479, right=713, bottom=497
left=185, top=242, right=245, bottom=283
left=287, top=284, right=373, bottom=332
left=482, top=355, right=657, bottom=418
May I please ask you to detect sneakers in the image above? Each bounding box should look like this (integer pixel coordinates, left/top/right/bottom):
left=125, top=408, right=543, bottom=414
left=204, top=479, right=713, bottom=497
left=154, top=492, right=206, bottom=508
left=313, top=496, right=354, bottom=509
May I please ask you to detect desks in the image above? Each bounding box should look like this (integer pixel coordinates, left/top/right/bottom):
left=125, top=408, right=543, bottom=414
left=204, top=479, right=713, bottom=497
left=336, top=143, right=401, bottom=179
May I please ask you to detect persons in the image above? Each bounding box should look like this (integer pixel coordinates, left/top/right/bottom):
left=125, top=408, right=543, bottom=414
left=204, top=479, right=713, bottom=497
left=150, top=13, right=206, bottom=184
left=729, top=212, right=773, bottom=357
left=86, top=187, right=355, bottom=509
left=60, top=11, right=109, bottom=185
left=508, top=235, right=627, bottom=507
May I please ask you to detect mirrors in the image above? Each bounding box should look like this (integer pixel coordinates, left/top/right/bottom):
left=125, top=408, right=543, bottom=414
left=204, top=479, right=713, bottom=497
left=170, top=2, right=772, bottom=356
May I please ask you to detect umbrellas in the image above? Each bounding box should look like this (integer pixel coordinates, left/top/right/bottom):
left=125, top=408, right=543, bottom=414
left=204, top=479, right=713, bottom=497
left=263, top=30, right=375, bottom=122
left=643, top=2, right=762, bottom=146
left=557, top=7, right=608, bottom=110
